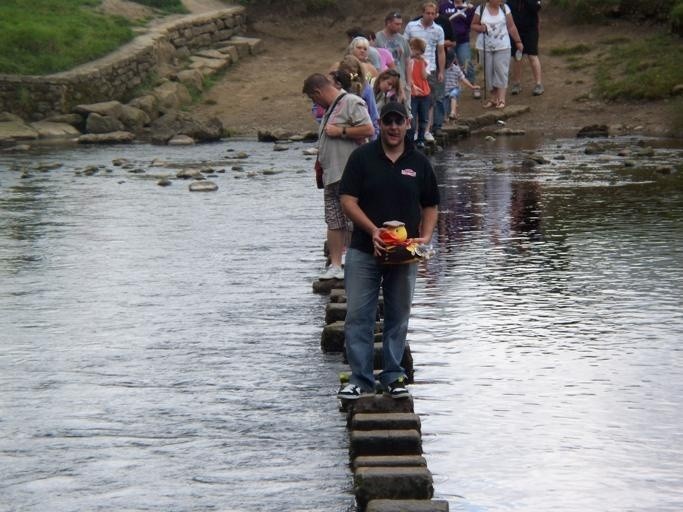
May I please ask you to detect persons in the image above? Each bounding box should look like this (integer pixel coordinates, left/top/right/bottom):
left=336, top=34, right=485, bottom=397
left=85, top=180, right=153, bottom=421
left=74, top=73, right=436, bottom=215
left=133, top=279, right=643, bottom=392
left=302, top=73, right=375, bottom=281
left=374, top=10, right=412, bottom=124
left=364, top=29, right=407, bottom=104
left=506, top=0, right=544, bottom=95
left=311, top=70, right=363, bottom=125
left=339, top=54, right=380, bottom=141
left=337, top=102, right=440, bottom=402
left=470, top=0, right=524, bottom=109
left=345, top=26, right=380, bottom=72
left=439, top=0, right=481, bottom=99
left=325, top=36, right=378, bottom=85
left=402, top=2, right=447, bottom=142
left=410, top=39, right=432, bottom=148
left=365, top=69, right=401, bottom=132
left=414, top=1, right=456, bottom=70
left=443, top=50, right=480, bottom=123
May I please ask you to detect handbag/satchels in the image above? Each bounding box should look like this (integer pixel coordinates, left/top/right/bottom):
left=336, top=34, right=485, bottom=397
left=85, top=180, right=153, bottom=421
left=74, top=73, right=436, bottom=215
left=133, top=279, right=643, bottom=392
left=314, top=160, right=323, bottom=189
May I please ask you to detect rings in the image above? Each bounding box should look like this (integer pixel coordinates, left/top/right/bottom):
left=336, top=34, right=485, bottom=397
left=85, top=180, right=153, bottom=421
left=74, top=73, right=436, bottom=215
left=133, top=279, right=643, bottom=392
left=373, top=246, right=376, bottom=247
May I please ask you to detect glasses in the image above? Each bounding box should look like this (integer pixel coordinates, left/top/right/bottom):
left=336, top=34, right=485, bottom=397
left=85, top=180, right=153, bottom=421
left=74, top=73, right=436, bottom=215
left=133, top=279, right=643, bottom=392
left=418, top=48, right=425, bottom=53
left=379, top=116, right=406, bottom=126
left=389, top=12, right=402, bottom=19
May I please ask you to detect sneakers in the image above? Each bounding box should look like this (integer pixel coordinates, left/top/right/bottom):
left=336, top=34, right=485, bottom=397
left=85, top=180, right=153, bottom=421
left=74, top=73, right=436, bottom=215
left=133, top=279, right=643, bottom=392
left=532, top=83, right=544, bottom=96
left=435, top=129, right=443, bottom=137
left=510, top=84, right=522, bottom=95
left=318, top=263, right=344, bottom=280
left=336, top=383, right=376, bottom=400
left=482, top=100, right=495, bottom=109
left=423, top=132, right=435, bottom=142
left=495, top=98, right=505, bottom=109
left=382, top=379, right=409, bottom=401
left=449, top=112, right=457, bottom=120
left=473, top=89, right=481, bottom=98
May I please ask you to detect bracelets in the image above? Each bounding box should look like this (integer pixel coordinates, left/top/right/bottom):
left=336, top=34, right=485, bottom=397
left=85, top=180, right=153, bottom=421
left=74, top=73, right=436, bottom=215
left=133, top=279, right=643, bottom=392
left=514, top=41, right=522, bottom=43
left=342, top=127, right=346, bottom=139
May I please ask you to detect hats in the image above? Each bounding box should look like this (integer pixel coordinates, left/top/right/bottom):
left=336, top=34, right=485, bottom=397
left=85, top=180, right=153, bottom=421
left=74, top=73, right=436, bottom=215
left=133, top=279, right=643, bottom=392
left=379, top=102, right=406, bottom=120
